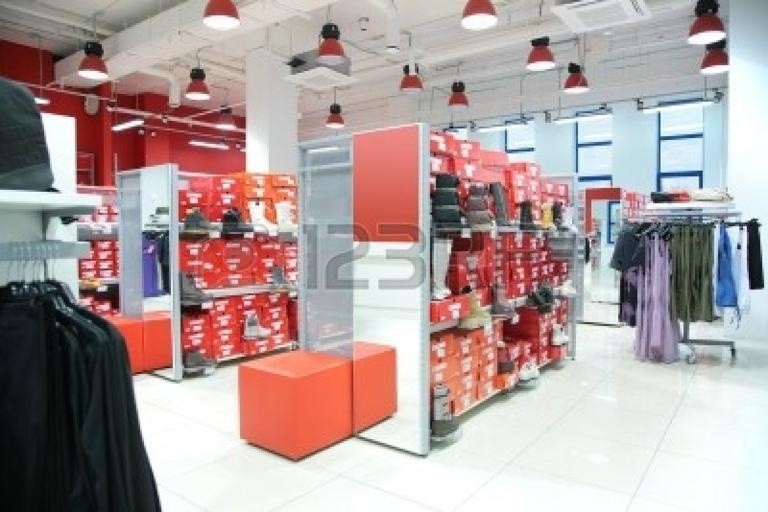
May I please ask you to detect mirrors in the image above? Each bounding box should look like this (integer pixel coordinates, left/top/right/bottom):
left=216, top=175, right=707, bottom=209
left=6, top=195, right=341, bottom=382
left=298, top=122, right=430, bottom=457
left=117, top=162, right=180, bottom=382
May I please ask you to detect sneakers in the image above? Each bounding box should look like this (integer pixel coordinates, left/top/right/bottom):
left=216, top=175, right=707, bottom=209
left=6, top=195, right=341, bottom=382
left=183, top=207, right=253, bottom=239
left=499, top=279, right=578, bottom=385
left=181, top=265, right=295, bottom=368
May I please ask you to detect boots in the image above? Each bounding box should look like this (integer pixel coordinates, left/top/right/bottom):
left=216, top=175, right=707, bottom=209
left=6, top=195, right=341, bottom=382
left=492, top=185, right=573, bottom=230
left=249, top=200, right=299, bottom=238
left=433, top=240, right=513, bottom=324
left=433, top=385, right=460, bottom=438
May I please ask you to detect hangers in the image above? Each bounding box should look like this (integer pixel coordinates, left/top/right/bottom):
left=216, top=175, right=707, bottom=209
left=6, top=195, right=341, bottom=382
left=1, top=240, right=124, bottom=362
left=618, top=215, right=760, bottom=253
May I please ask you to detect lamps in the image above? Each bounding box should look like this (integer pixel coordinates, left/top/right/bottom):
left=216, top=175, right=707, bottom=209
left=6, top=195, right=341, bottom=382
left=461, top=0, right=592, bottom=95
left=183, top=0, right=244, bottom=102
left=686, top=0, right=729, bottom=78
left=76, top=13, right=109, bottom=81
left=315, top=3, right=469, bottom=129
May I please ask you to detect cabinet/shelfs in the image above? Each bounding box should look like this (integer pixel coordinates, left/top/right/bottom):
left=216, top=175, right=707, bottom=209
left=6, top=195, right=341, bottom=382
left=431, top=169, right=580, bottom=452
left=179, top=174, right=298, bottom=380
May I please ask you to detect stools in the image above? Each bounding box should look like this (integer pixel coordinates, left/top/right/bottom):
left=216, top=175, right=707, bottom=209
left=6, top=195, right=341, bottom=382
left=144, top=311, right=172, bottom=371
left=237, top=349, right=355, bottom=461
left=105, top=318, right=144, bottom=375
left=344, top=341, right=397, bottom=435
